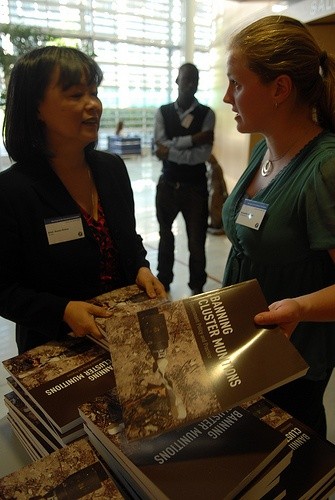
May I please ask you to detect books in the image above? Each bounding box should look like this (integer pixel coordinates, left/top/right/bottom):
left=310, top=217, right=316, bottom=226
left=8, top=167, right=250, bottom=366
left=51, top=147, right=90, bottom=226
left=0, top=277, right=335, bottom=500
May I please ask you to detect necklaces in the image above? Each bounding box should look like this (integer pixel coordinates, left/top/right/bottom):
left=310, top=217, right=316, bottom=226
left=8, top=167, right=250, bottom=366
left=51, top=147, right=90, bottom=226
left=261, top=142, right=297, bottom=176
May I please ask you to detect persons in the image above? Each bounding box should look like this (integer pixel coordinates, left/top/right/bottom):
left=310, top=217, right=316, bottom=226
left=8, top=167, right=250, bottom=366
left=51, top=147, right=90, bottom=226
left=154, top=63, right=216, bottom=297
left=220, top=15, right=335, bottom=440
left=0, top=45, right=169, bottom=355
